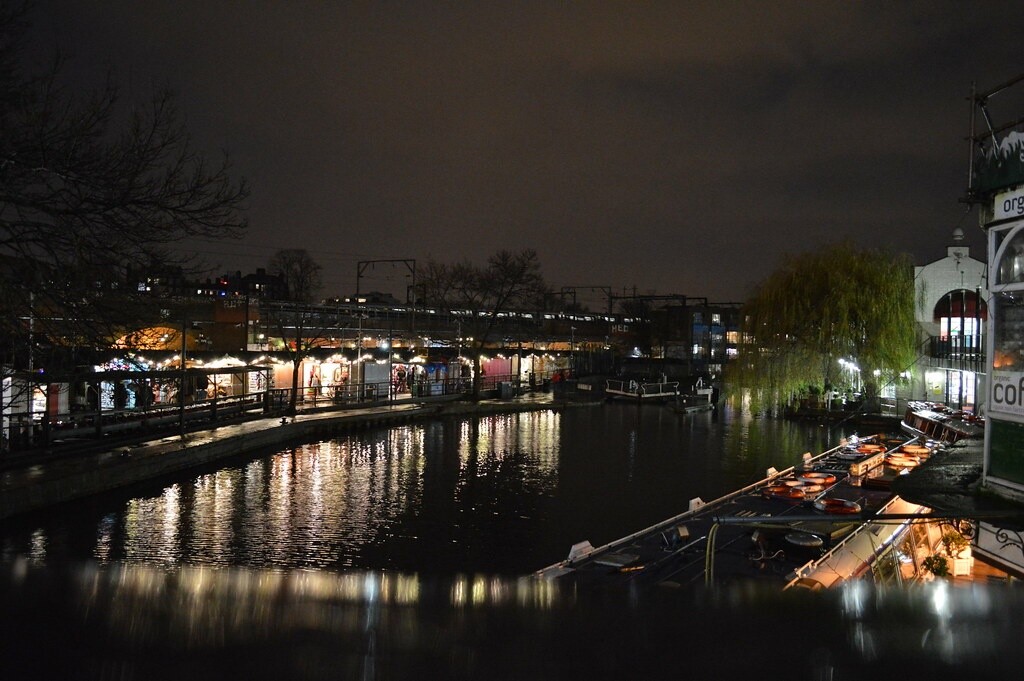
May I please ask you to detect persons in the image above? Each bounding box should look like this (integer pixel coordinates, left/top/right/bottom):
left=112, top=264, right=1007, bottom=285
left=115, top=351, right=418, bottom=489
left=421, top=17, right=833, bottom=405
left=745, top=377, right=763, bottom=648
left=459, top=358, right=474, bottom=381
left=845, top=389, right=853, bottom=406
left=392, top=365, right=423, bottom=393
left=308, top=371, right=322, bottom=396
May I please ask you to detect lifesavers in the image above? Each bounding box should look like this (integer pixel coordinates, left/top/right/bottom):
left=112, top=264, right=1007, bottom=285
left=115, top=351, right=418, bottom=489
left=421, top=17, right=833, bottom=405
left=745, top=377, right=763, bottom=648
left=797, top=472, right=836, bottom=485
left=784, top=532, right=823, bottom=547
left=762, top=486, right=806, bottom=499
left=888, top=439, right=904, bottom=443
left=619, top=566, right=647, bottom=575
left=783, top=481, right=821, bottom=493
left=836, top=449, right=866, bottom=460
left=815, top=498, right=862, bottom=515
left=885, top=453, right=920, bottom=466
left=900, top=445, right=931, bottom=454
left=856, top=444, right=888, bottom=454
